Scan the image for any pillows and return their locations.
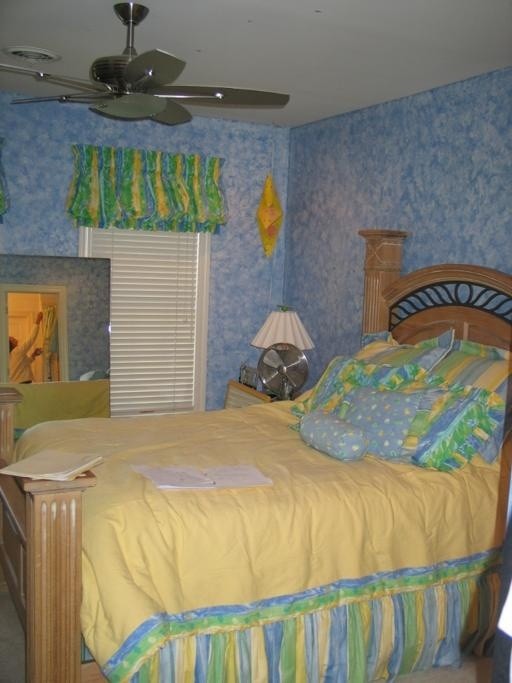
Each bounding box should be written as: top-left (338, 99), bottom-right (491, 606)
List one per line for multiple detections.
top-left (295, 328), bottom-right (511, 474)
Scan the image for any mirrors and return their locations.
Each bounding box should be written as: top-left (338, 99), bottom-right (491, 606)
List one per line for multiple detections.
top-left (0, 283), bottom-right (69, 384)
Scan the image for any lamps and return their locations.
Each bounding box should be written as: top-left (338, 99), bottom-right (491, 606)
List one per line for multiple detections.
top-left (249, 305), bottom-right (316, 353)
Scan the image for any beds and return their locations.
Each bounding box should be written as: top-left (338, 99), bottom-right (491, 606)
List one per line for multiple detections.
top-left (0, 229), bottom-right (511, 683)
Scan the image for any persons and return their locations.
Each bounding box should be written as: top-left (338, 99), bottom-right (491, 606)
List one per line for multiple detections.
top-left (7, 312), bottom-right (44, 386)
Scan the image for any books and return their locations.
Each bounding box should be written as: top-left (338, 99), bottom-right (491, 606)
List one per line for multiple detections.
top-left (0, 447), bottom-right (104, 483)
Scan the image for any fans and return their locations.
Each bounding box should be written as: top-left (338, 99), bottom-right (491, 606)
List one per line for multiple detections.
top-left (0, 3), bottom-right (292, 127)
top-left (256, 343), bottom-right (308, 398)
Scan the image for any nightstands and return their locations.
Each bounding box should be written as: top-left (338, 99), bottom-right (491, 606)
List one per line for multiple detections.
top-left (222, 381), bottom-right (302, 410)
